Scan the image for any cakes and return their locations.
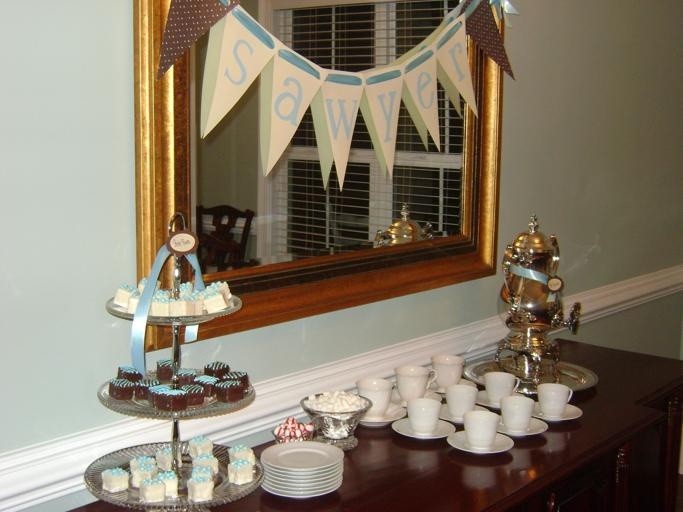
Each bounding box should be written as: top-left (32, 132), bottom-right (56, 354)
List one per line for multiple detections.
top-left (274, 418), bottom-right (310, 443)
top-left (108, 360), bottom-right (249, 411)
top-left (100, 436), bottom-right (219, 503)
top-left (228, 459), bottom-right (254, 485)
top-left (228, 445), bottom-right (256, 465)
top-left (114, 279), bottom-right (232, 316)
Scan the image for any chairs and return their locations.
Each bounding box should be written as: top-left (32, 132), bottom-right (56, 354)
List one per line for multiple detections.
top-left (196, 204), bottom-right (256, 276)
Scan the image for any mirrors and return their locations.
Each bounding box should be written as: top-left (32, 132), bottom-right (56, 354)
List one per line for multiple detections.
top-left (132, 0), bottom-right (506, 352)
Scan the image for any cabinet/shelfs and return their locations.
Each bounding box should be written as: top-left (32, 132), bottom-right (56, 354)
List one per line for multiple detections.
top-left (62, 336), bottom-right (682, 512)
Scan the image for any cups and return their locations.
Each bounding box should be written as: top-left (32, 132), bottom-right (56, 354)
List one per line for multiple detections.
top-left (351, 354), bottom-right (584, 457)
top-left (297, 390), bottom-right (373, 453)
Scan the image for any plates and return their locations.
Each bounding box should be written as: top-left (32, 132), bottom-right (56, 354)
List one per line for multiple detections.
top-left (258, 438), bottom-right (346, 501)
